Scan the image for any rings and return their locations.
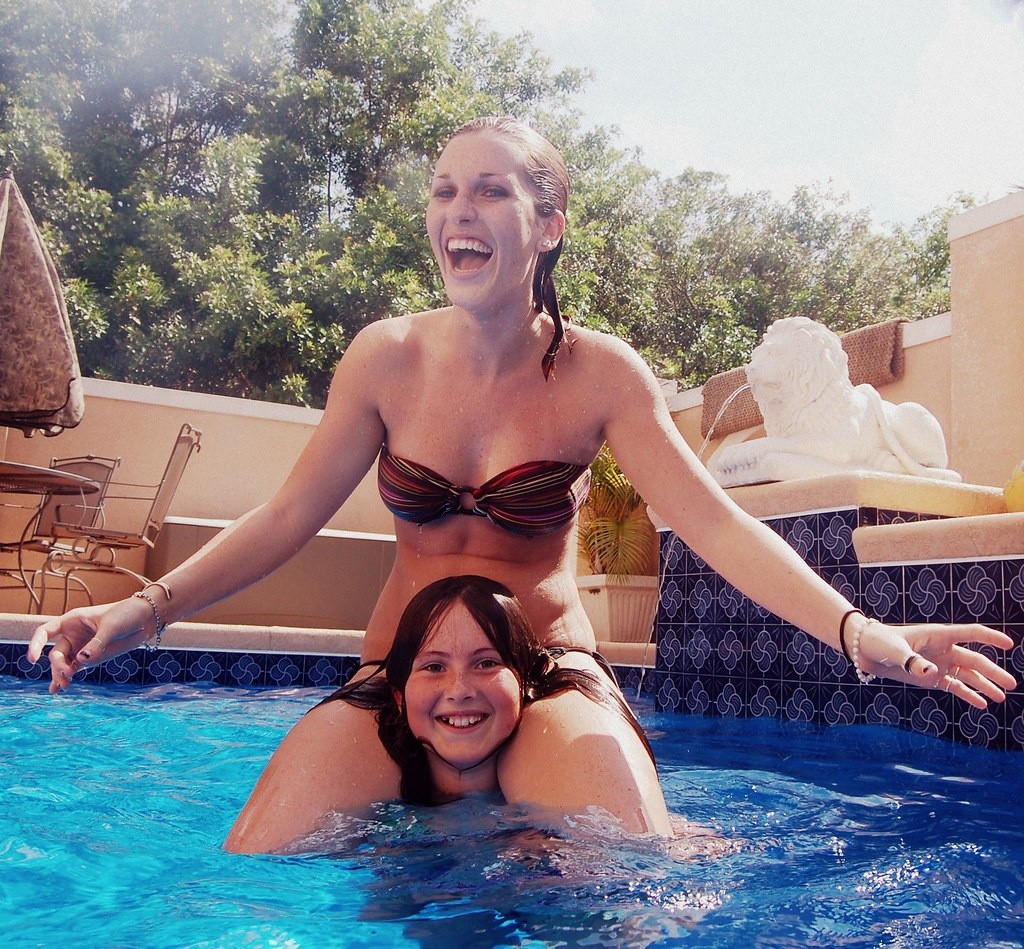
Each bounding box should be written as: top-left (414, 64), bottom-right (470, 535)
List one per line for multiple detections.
top-left (91, 637), bottom-right (106, 653)
top-left (900, 651), bottom-right (922, 675)
top-left (953, 664), bottom-right (962, 680)
top-left (946, 678), bottom-right (955, 692)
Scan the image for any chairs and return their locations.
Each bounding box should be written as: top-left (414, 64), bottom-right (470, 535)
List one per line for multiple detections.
top-left (0, 453), bottom-right (121, 614)
top-left (35, 423), bottom-right (204, 618)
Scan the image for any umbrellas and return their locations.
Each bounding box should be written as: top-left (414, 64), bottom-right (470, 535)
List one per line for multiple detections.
top-left (1, 174), bottom-right (86, 437)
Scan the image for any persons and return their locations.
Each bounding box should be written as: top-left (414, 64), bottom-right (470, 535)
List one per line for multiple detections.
top-left (305, 576), bottom-right (656, 797)
top-left (26, 105), bottom-right (1016, 853)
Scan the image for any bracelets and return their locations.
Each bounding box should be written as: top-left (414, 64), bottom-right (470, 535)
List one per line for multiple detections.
top-left (853, 617), bottom-right (881, 683)
top-left (840, 607), bottom-right (864, 664)
top-left (130, 590), bottom-right (160, 652)
top-left (142, 581), bottom-right (172, 634)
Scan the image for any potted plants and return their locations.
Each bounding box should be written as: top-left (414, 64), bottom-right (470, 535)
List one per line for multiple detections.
top-left (578, 442), bottom-right (660, 644)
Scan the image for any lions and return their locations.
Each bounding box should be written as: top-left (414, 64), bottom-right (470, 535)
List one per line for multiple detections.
top-left (744, 316), bottom-right (965, 481)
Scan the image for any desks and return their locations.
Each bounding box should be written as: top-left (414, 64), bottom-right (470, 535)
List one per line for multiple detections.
top-left (0, 460), bottom-right (101, 615)
